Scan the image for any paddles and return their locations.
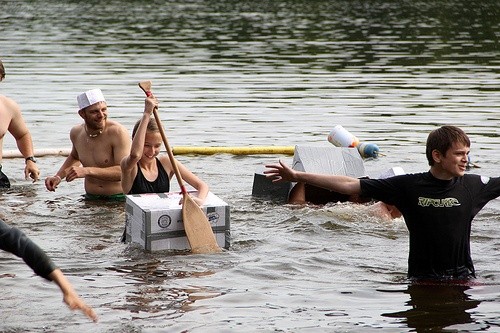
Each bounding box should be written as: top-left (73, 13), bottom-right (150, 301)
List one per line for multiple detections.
top-left (138, 81), bottom-right (221, 252)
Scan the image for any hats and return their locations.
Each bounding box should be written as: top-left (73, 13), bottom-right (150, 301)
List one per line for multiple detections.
top-left (76, 89), bottom-right (105, 111)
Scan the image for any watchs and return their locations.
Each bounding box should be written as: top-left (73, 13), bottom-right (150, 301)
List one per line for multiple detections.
top-left (26, 157), bottom-right (37, 163)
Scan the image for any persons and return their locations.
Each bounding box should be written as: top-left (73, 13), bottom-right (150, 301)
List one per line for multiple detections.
top-left (0, 218), bottom-right (98, 322)
top-left (287, 180), bottom-right (403, 220)
top-left (264, 126), bottom-right (500, 281)
top-left (0, 58), bottom-right (39, 192)
top-left (118, 95), bottom-right (209, 243)
top-left (45, 89), bottom-right (131, 204)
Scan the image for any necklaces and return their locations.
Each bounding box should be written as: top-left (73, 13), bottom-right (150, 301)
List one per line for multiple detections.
top-left (84, 120), bottom-right (105, 137)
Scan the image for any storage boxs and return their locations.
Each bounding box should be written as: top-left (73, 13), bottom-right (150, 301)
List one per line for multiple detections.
top-left (126, 191), bottom-right (230, 252)
top-left (380, 167), bottom-right (404, 179)
top-left (252, 173), bottom-right (299, 201)
top-left (292, 146), bottom-right (370, 203)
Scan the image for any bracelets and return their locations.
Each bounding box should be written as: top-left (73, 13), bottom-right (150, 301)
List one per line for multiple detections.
top-left (54, 175), bottom-right (62, 182)
